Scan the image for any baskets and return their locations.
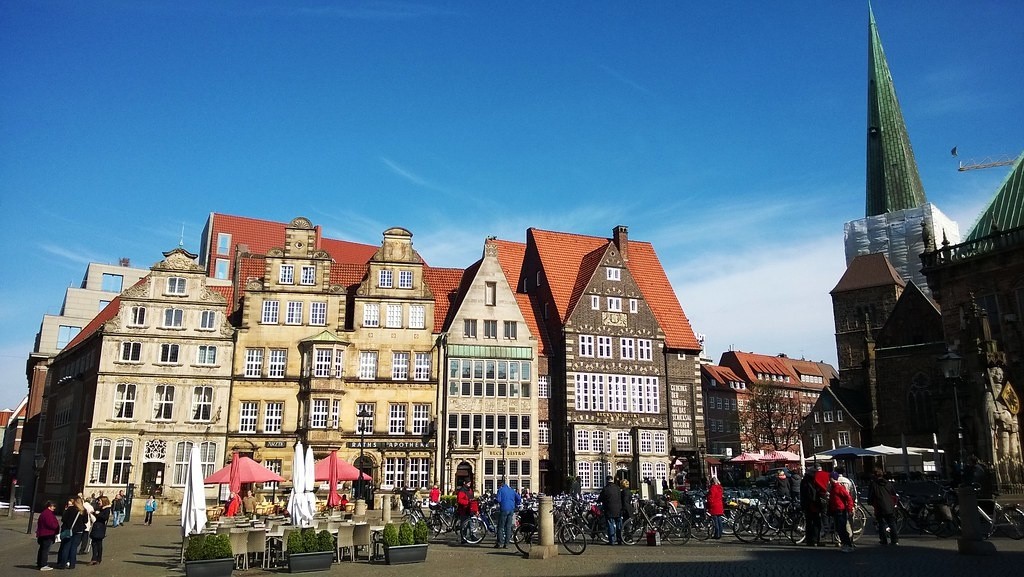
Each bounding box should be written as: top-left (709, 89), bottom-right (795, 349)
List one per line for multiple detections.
top-left (428, 503), bottom-right (441, 510)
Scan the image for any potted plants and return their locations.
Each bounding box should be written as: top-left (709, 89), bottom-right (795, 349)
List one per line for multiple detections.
top-left (382, 520), bottom-right (429, 565)
top-left (284, 528), bottom-right (334, 572)
top-left (184, 533), bottom-right (235, 577)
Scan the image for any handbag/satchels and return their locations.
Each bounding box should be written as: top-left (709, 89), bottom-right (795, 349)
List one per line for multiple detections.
top-left (815, 496), bottom-right (827, 513)
top-left (845, 504), bottom-right (855, 519)
top-left (60, 529), bottom-right (72, 540)
top-left (470, 501), bottom-right (478, 511)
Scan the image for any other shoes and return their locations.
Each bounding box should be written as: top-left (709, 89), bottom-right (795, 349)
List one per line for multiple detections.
top-left (625, 540), bottom-right (636, 544)
top-left (879, 541), bottom-right (899, 546)
top-left (711, 536), bottom-right (721, 540)
top-left (88, 560), bottom-right (101, 566)
top-left (493, 544), bottom-right (507, 548)
top-left (78, 551), bottom-right (87, 555)
top-left (55, 562), bottom-right (75, 569)
top-left (113, 523), bottom-right (124, 528)
top-left (461, 536), bottom-right (478, 544)
top-left (607, 541), bottom-right (622, 546)
top-left (40, 565), bottom-right (53, 571)
top-left (144, 523), bottom-right (151, 526)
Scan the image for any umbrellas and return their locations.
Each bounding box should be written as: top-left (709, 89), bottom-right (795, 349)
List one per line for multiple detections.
top-left (805, 443), bottom-right (923, 478)
top-left (180, 436), bottom-right (373, 571)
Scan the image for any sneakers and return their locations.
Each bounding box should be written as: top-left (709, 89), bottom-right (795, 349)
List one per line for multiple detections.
top-left (840, 543), bottom-right (855, 553)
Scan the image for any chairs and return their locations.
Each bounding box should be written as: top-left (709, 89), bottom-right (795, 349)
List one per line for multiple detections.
top-left (854, 471), bottom-right (937, 484)
top-left (181, 502), bottom-right (382, 571)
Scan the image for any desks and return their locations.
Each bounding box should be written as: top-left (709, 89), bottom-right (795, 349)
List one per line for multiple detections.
top-left (266, 526), bottom-right (384, 563)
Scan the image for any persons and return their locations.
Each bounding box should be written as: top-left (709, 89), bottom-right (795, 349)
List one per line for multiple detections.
top-left (489, 481), bottom-right (520, 550)
top-left (400, 486), bottom-right (411, 504)
top-left (243, 488), bottom-right (256, 517)
top-left (706, 478), bottom-right (725, 540)
top-left (775, 462), bottom-right (902, 552)
top-left (596, 476), bottom-right (623, 546)
top-left (619, 479), bottom-right (634, 546)
top-left (457, 477), bottom-right (480, 544)
top-left (341, 495), bottom-right (349, 512)
top-left (430, 485), bottom-right (439, 504)
top-left (985, 367), bottom-right (1023, 484)
top-left (36, 490), bottom-right (156, 572)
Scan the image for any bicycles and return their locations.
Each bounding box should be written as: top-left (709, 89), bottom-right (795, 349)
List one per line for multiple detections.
top-left (399, 471), bottom-right (1024, 548)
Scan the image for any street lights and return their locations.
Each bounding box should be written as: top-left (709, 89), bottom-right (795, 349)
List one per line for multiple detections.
top-left (26, 453), bottom-right (47, 534)
top-left (356, 403), bottom-right (373, 499)
top-left (936, 345), bottom-right (965, 482)
top-left (599, 450), bottom-right (606, 486)
top-left (699, 442), bottom-right (708, 489)
top-left (500, 432), bottom-right (509, 482)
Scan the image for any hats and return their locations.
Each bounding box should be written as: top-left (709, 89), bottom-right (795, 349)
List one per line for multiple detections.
top-left (620, 479), bottom-right (629, 488)
top-left (830, 471), bottom-right (839, 480)
top-left (779, 471), bottom-right (784, 476)
